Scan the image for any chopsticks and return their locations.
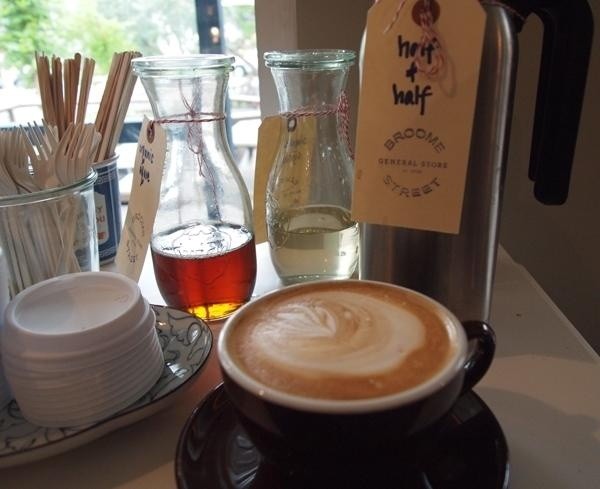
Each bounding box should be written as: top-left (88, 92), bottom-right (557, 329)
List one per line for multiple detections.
top-left (33, 50), bottom-right (141, 164)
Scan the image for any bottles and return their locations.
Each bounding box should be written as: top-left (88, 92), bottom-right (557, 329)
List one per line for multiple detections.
top-left (357, 1), bottom-right (514, 323)
top-left (129, 56), bottom-right (256, 323)
top-left (263, 50), bottom-right (363, 285)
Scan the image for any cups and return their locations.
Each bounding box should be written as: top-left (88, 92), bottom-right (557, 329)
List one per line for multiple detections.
top-left (216, 277), bottom-right (497, 487)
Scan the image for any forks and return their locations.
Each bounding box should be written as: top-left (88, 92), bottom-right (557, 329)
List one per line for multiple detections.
top-left (0, 118), bottom-right (101, 297)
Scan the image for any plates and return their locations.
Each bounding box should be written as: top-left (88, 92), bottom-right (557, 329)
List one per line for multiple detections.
top-left (1, 300), bottom-right (212, 472)
top-left (0, 271), bottom-right (166, 429)
top-left (173, 380), bottom-right (503, 486)
